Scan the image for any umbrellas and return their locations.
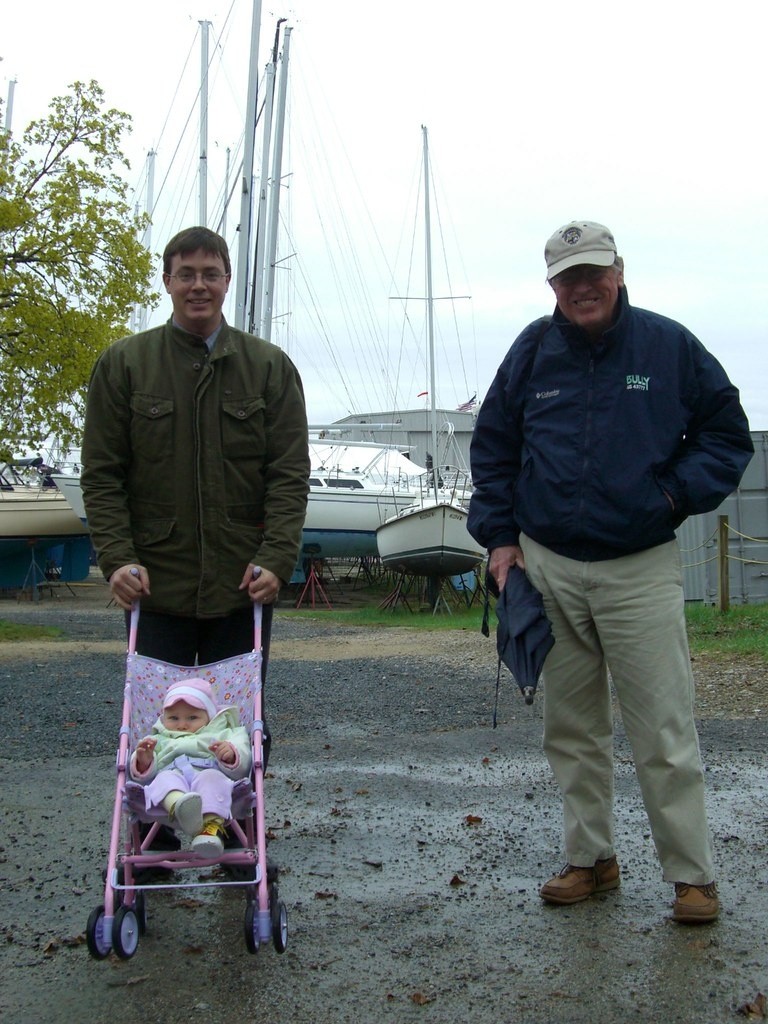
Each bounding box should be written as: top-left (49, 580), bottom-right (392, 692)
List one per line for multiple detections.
top-left (483, 554), bottom-right (554, 703)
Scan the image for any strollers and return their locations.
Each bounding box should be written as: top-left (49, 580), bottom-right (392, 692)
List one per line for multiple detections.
top-left (84, 560), bottom-right (292, 959)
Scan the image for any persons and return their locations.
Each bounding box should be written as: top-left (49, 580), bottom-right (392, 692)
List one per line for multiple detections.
top-left (466, 220), bottom-right (756, 923)
top-left (130, 678), bottom-right (251, 859)
top-left (80, 227), bottom-right (311, 887)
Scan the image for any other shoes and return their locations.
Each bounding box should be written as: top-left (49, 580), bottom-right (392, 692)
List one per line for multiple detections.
top-left (102, 863), bottom-right (159, 886)
top-left (191, 834), bottom-right (222, 857)
top-left (175, 791), bottom-right (204, 836)
top-left (220, 848), bottom-right (278, 883)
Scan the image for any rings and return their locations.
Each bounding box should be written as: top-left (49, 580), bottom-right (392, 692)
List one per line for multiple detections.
top-left (264, 597), bottom-right (267, 600)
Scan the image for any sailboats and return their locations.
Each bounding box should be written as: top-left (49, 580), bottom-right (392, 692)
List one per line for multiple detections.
top-left (371, 115), bottom-right (490, 585)
top-left (0, 0), bottom-right (454, 569)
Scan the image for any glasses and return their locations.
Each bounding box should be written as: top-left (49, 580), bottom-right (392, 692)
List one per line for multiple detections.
top-left (167, 269), bottom-right (229, 285)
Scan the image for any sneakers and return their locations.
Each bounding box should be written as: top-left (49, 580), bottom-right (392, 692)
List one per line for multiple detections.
top-left (538, 853), bottom-right (620, 903)
top-left (672, 880), bottom-right (720, 922)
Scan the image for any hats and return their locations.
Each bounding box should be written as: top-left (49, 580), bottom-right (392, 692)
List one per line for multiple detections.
top-left (545, 220), bottom-right (617, 281)
top-left (163, 678), bottom-right (218, 722)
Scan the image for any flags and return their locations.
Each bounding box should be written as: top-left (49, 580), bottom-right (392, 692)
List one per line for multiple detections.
top-left (458, 395), bottom-right (476, 412)
